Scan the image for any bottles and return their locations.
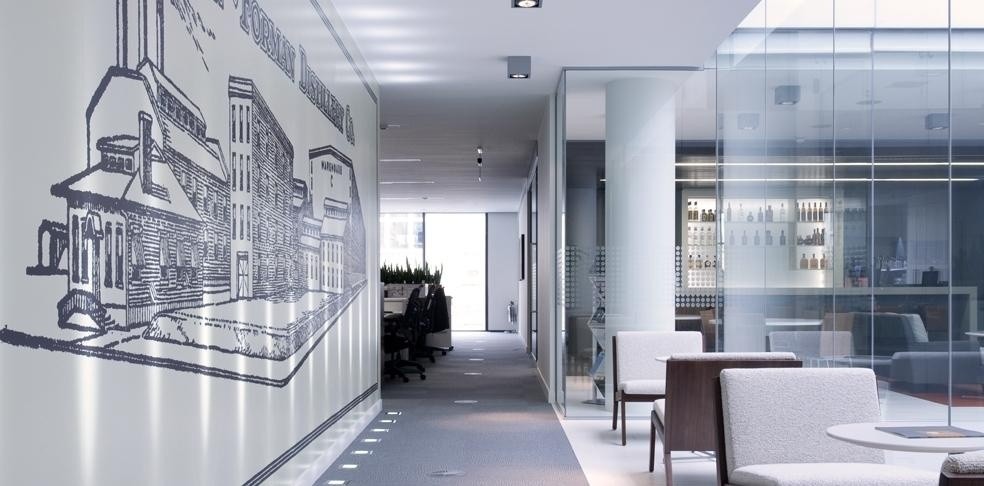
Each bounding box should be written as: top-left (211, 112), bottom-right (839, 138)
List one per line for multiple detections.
top-left (737, 202), bottom-right (787, 223)
top-left (780, 230), bottom-right (787, 245)
top-left (726, 201), bottom-right (732, 221)
top-left (765, 230), bottom-right (773, 246)
top-left (797, 228), bottom-right (825, 247)
top-left (796, 202), bottom-right (831, 222)
top-left (688, 254), bottom-right (717, 269)
top-left (741, 230), bottom-right (748, 245)
top-left (688, 226), bottom-right (717, 245)
top-left (687, 201), bottom-right (718, 222)
top-left (799, 253), bottom-right (828, 269)
top-left (728, 228), bottom-right (736, 246)
top-left (753, 230), bottom-right (761, 245)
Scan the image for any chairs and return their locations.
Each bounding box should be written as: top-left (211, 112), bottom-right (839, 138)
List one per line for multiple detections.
top-left (380, 284), bottom-right (454, 382)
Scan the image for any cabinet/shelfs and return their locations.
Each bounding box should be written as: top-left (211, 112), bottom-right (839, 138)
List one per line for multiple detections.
top-left (681, 188), bottom-right (844, 286)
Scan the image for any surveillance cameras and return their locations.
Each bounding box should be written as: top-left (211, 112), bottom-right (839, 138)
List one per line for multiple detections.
top-left (476, 155), bottom-right (483, 166)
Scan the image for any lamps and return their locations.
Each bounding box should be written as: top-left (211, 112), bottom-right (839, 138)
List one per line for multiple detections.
top-left (775, 86), bottom-right (800, 105)
top-left (924, 114), bottom-right (949, 131)
top-left (738, 115), bottom-right (758, 130)
top-left (507, 56), bottom-right (531, 79)
top-left (511, 0), bottom-right (542, 8)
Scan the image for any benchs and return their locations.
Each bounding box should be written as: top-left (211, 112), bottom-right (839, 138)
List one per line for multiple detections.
top-left (611, 309), bottom-right (984, 486)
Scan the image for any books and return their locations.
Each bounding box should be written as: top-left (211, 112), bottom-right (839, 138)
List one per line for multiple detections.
top-left (875, 425), bottom-right (984, 439)
